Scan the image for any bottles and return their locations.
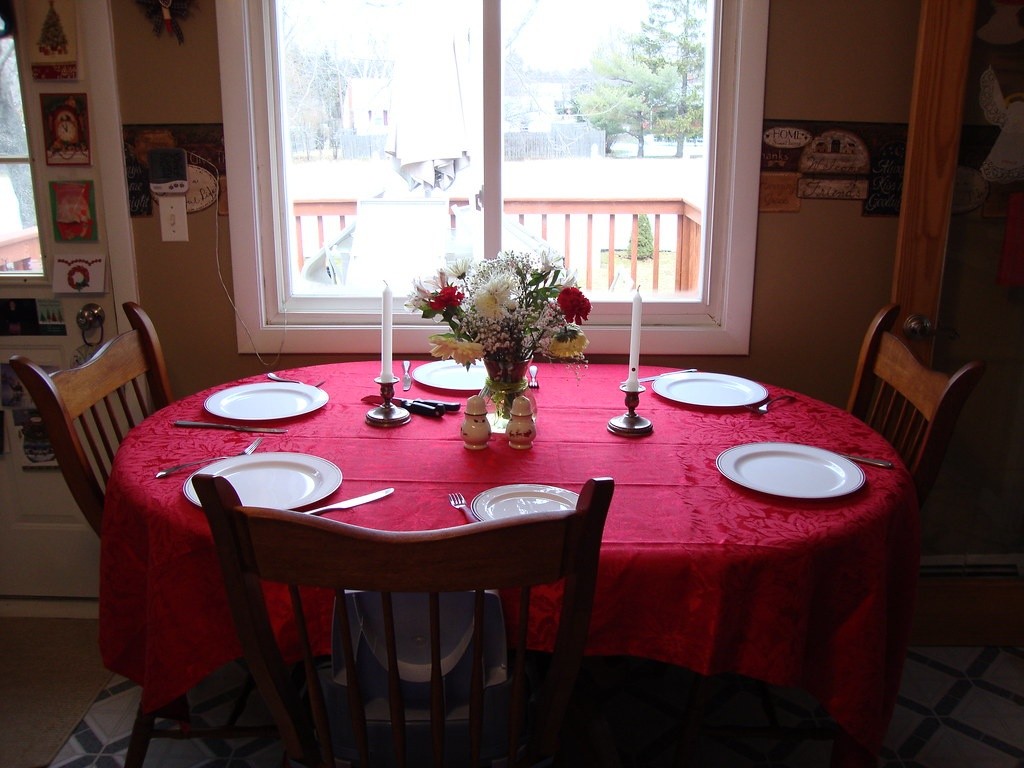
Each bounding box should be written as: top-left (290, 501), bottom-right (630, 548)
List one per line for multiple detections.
top-left (460, 396), bottom-right (491, 450)
top-left (506, 397), bottom-right (536, 450)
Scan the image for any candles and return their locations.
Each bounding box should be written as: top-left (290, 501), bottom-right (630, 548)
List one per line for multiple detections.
top-left (628, 286), bottom-right (641, 391)
top-left (380, 280), bottom-right (393, 382)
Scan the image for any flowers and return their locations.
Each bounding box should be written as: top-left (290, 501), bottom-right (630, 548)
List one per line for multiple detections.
top-left (402, 246), bottom-right (591, 420)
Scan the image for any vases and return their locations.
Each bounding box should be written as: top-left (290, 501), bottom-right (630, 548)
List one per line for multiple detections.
top-left (478, 377), bottom-right (538, 433)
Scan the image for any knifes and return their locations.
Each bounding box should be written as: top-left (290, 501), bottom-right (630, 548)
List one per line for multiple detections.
top-left (304, 488), bottom-right (394, 515)
top-left (620, 369), bottom-right (698, 384)
top-left (175, 421), bottom-right (288, 432)
top-left (403, 361), bottom-right (411, 390)
top-left (361, 395), bottom-right (460, 418)
top-left (825, 449), bottom-right (893, 469)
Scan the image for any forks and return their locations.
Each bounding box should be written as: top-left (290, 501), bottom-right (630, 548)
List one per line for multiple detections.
top-left (155, 438), bottom-right (263, 478)
top-left (268, 373), bottom-right (325, 387)
top-left (449, 493), bottom-right (479, 523)
top-left (745, 396), bottom-right (795, 414)
top-left (530, 366), bottom-right (538, 387)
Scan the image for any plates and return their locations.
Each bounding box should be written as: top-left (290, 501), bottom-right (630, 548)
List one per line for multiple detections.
top-left (204, 382), bottom-right (329, 421)
top-left (183, 452), bottom-right (342, 510)
top-left (716, 443), bottom-right (866, 498)
top-left (471, 484), bottom-right (579, 522)
top-left (412, 358), bottom-right (489, 390)
top-left (652, 372), bottom-right (768, 406)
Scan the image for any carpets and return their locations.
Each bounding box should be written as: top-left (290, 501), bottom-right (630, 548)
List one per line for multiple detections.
top-left (0, 617), bottom-right (118, 768)
top-left (46, 641), bottom-right (1024, 768)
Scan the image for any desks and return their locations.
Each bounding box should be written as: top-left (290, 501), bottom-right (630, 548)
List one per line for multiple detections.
top-left (100, 360), bottom-right (922, 768)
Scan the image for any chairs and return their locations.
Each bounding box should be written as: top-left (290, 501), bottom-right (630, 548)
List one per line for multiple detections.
top-left (9, 303), bottom-right (313, 768)
top-left (192, 474), bottom-right (615, 768)
top-left (848, 303), bottom-right (983, 500)
